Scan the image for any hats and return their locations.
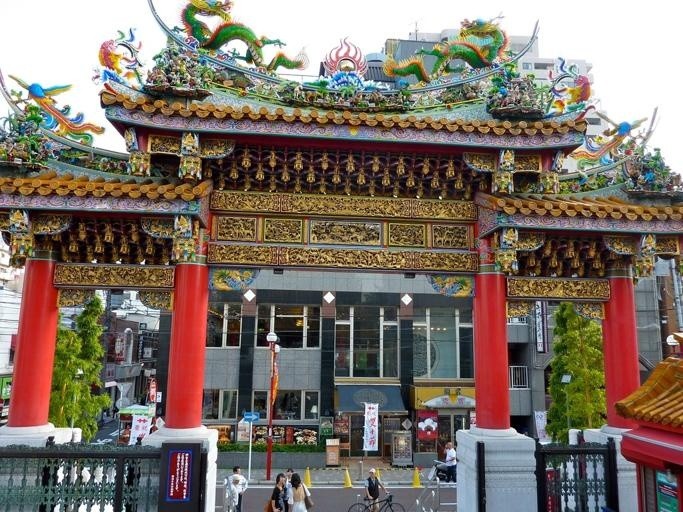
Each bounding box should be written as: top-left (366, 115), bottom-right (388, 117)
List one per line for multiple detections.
top-left (369, 468), bottom-right (376, 473)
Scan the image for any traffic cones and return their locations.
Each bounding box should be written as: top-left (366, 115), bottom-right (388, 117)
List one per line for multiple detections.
top-left (411, 465), bottom-right (422, 488)
top-left (344, 467), bottom-right (352, 488)
top-left (375, 467), bottom-right (380, 477)
top-left (303, 466), bottom-right (313, 488)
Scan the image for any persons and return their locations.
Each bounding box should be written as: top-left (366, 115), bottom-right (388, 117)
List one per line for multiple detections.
top-left (272, 468), bottom-right (311, 512)
top-left (443, 442), bottom-right (456, 482)
top-left (364, 468), bottom-right (388, 512)
top-left (122, 424), bottom-right (131, 441)
top-left (224, 466), bottom-right (248, 512)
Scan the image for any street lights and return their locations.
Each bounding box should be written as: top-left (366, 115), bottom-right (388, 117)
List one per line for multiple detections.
top-left (104, 381), bottom-right (123, 439)
top-left (266, 330), bottom-right (280, 480)
top-left (665, 334), bottom-right (679, 354)
top-left (560, 373), bottom-right (572, 430)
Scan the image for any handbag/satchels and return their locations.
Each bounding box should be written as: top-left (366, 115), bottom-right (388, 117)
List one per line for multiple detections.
top-left (264, 499), bottom-right (273, 512)
top-left (305, 496), bottom-right (314, 508)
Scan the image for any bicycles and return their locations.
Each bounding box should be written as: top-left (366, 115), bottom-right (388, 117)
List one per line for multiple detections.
top-left (347, 492), bottom-right (406, 511)
